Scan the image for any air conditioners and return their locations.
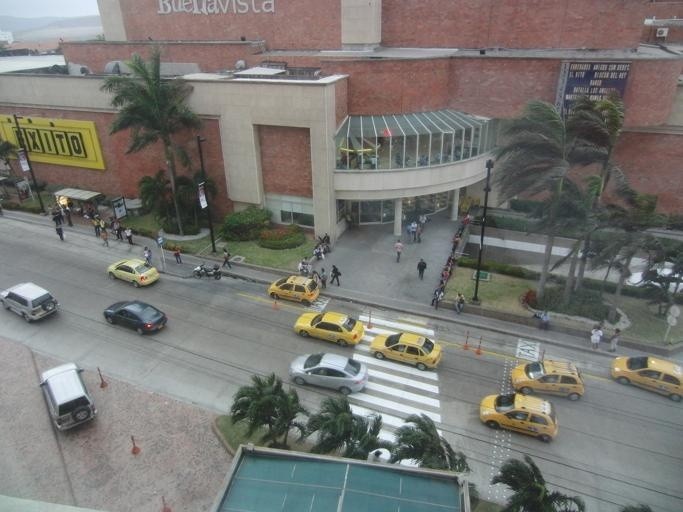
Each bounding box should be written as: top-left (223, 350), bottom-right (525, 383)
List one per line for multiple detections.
top-left (655, 27), bottom-right (669, 38)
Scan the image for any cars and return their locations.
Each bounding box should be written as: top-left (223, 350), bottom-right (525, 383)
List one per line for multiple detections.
top-left (104, 299), bottom-right (167, 337)
top-left (292, 311), bottom-right (365, 346)
top-left (513, 359), bottom-right (586, 401)
top-left (614, 355), bottom-right (683, 401)
top-left (478, 391), bottom-right (560, 444)
top-left (371, 332), bottom-right (442, 372)
top-left (266, 276), bottom-right (319, 304)
top-left (289, 352), bottom-right (368, 396)
top-left (624, 266), bottom-right (683, 295)
top-left (108, 260), bottom-right (158, 289)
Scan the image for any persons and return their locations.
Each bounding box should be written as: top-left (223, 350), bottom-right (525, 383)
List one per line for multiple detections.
top-left (539, 310), bottom-right (550, 330)
top-left (143, 246), bottom-right (153, 266)
top-left (396, 153), bottom-right (402, 166)
top-left (420, 154), bottom-right (425, 166)
top-left (221, 248), bottom-right (233, 269)
top-left (607, 327), bottom-right (621, 352)
top-left (173, 245), bottom-right (183, 264)
top-left (393, 212), bottom-right (470, 315)
top-left (50, 201), bottom-right (134, 249)
top-left (589, 325), bottom-right (604, 350)
top-left (341, 147), bottom-right (379, 169)
top-left (298, 232), bottom-right (342, 289)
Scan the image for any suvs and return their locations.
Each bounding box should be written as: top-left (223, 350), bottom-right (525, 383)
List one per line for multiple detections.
top-left (41, 363), bottom-right (98, 436)
top-left (0, 280), bottom-right (60, 323)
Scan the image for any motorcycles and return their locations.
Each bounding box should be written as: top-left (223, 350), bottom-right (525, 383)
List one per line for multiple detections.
top-left (193, 261), bottom-right (223, 280)
